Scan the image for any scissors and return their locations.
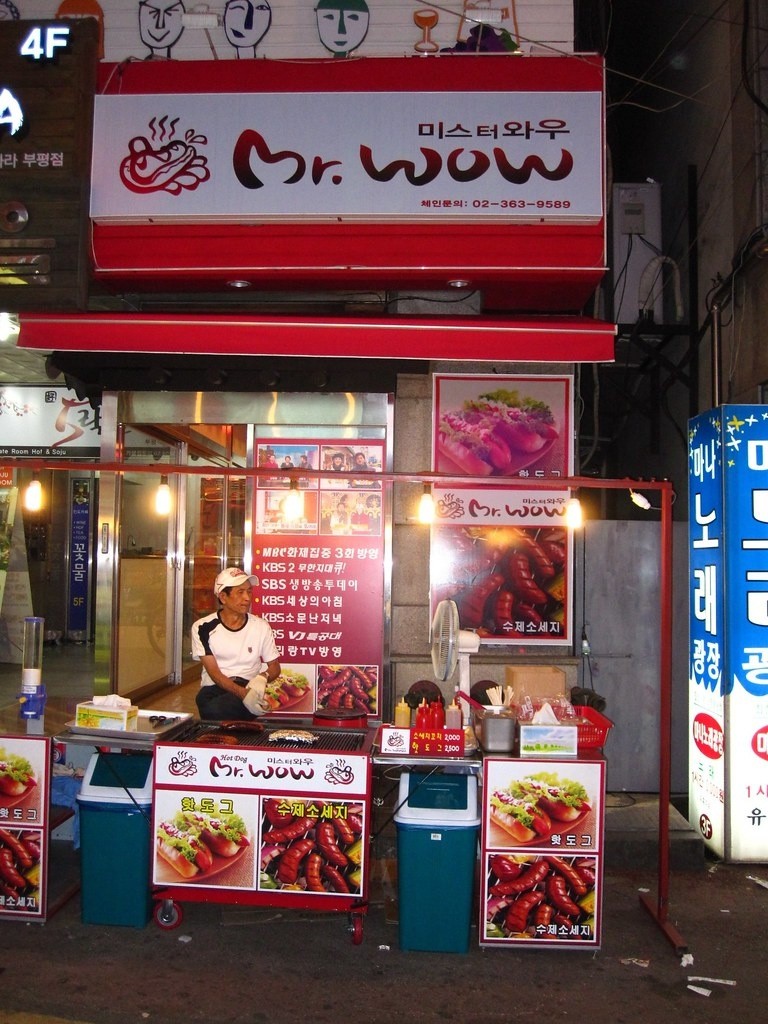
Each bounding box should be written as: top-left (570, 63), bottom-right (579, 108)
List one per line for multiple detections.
top-left (149, 715), bottom-right (166, 729)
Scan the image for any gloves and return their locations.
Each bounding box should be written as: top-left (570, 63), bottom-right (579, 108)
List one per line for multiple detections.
top-left (245, 675), bottom-right (267, 698)
top-left (242, 689), bottom-right (272, 716)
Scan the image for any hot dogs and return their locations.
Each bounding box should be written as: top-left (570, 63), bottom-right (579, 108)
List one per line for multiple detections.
top-left (489, 772), bottom-right (593, 842)
top-left (436, 389), bottom-right (558, 476)
top-left (0, 747), bottom-right (39, 797)
top-left (260, 669), bottom-right (311, 711)
top-left (156, 811), bottom-right (250, 877)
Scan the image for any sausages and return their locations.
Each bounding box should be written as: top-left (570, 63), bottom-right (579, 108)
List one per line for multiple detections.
top-left (316, 664), bottom-right (376, 714)
top-left (439, 530), bottom-right (568, 637)
top-left (488, 854), bottom-right (595, 939)
top-left (0, 828), bottom-right (32, 910)
top-left (260, 802), bottom-right (353, 894)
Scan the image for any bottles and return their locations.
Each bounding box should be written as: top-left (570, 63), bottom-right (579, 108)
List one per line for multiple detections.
top-left (446, 699), bottom-right (461, 729)
top-left (394, 696), bottom-right (411, 728)
top-left (431, 693), bottom-right (444, 729)
top-left (414, 698), bottom-right (431, 728)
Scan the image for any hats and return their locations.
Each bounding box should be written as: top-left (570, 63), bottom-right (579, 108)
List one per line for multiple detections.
top-left (214, 567), bottom-right (260, 598)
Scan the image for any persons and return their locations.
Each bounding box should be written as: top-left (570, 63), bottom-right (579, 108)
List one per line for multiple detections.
top-left (350, 453), bottom-right (376, 471)
top-left (264, 455), bottom-right (278, 468)
top-left (351, 505), bottom-right (369, 534)
top-left (191, 568), bottom-right (281, 721)
top-left (329, 504), bottom-right (350, 534)
top-left (281, 456), bottom-right (294, 468)
top-left (326, 451), bottom-right (348, 471)
top-left (299, 455), bottom-right (312, 470)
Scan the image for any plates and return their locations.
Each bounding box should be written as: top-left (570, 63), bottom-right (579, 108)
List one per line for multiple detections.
top-left (158, 820), bottom-right (247, 882)
top-left (495, 791), bottom-right (592, 847)
top-left (270, 687), bottom-right (310, 710)
top-left (0, 770), bottom-right (37, 808)
top-left (441, 404), bottom-right (557, 477)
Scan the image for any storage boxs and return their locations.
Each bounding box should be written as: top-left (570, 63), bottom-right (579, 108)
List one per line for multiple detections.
top-left (505, 663), bottom-right (567, 705)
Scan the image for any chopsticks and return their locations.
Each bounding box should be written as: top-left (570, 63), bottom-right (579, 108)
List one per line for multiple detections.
top-left (486, 685), bottom-right (514, 705)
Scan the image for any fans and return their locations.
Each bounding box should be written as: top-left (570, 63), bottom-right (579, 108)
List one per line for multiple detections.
top-left (429, 599), bottom-right (482, 727)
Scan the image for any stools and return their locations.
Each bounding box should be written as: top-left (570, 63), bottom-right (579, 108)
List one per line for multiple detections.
top-left (311, 707), bottom-right (369, 728)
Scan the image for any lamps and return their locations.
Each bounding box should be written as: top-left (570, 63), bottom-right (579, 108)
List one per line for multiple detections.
top-left (582, 631), bottom-right (591, 657)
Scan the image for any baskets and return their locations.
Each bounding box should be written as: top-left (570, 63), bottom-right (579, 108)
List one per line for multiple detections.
top-left (512, 703), bottom-right (614, 747)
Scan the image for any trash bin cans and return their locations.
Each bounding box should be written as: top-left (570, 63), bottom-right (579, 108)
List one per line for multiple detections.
top-left (394, 771), bottom-right (482, 955)
top-left (74, 752), bottom-right (153, 929)
